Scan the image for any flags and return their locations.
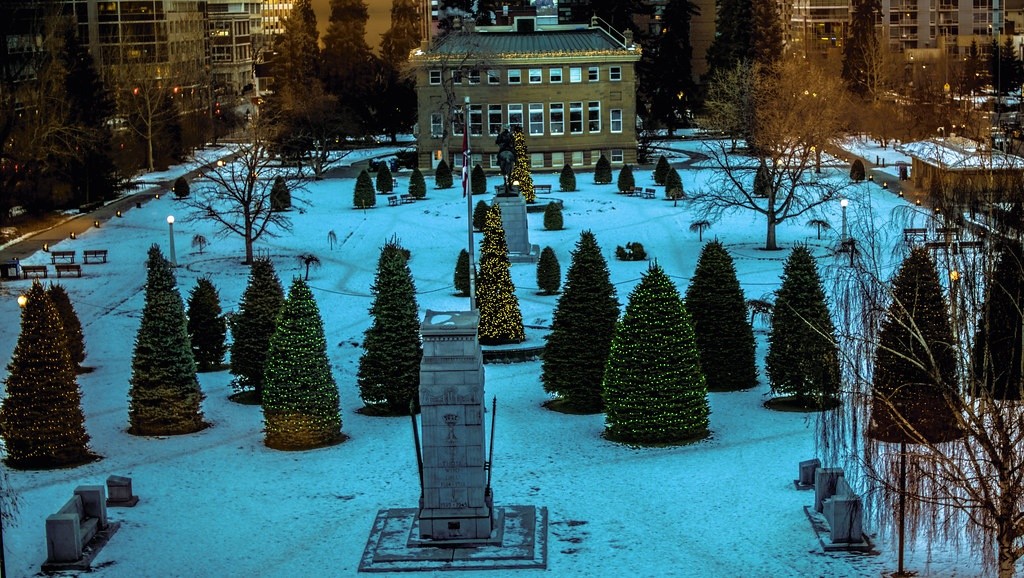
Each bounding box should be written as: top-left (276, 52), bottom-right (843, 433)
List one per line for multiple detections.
top-left (460, 97), bottom-right (470, 200)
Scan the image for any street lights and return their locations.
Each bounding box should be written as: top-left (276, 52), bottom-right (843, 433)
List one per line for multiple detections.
top-left (840, 198), bottom-right (848, 242)
top-left (167, 215), bottom-right (178, 266)
top-left (17, 296), bottom-right (27, 317)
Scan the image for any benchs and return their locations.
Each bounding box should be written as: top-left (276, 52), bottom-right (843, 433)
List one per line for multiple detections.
top-left (55, 264), bottom-right (82, 277)
top-left (22, 266), bottom-right (47, 279)
top-left (401, 194), bottom-right (416, 203)
top-left (642, 188), bottom-right (655, 199)
top-left (625, 186), bottom-right (642, 197)
top-left (388, 196), bottom-right (400, 205)
top-left (82, 250), bottom-right (107, 264)
top-left (805, 467), bottom-right (871, 552)
top-left (41, 484), bottom-right (120, 574)
top-left (533, 184), bottom-right (551, 194)
top-left (51, 251), bottom-right (75, 264)
